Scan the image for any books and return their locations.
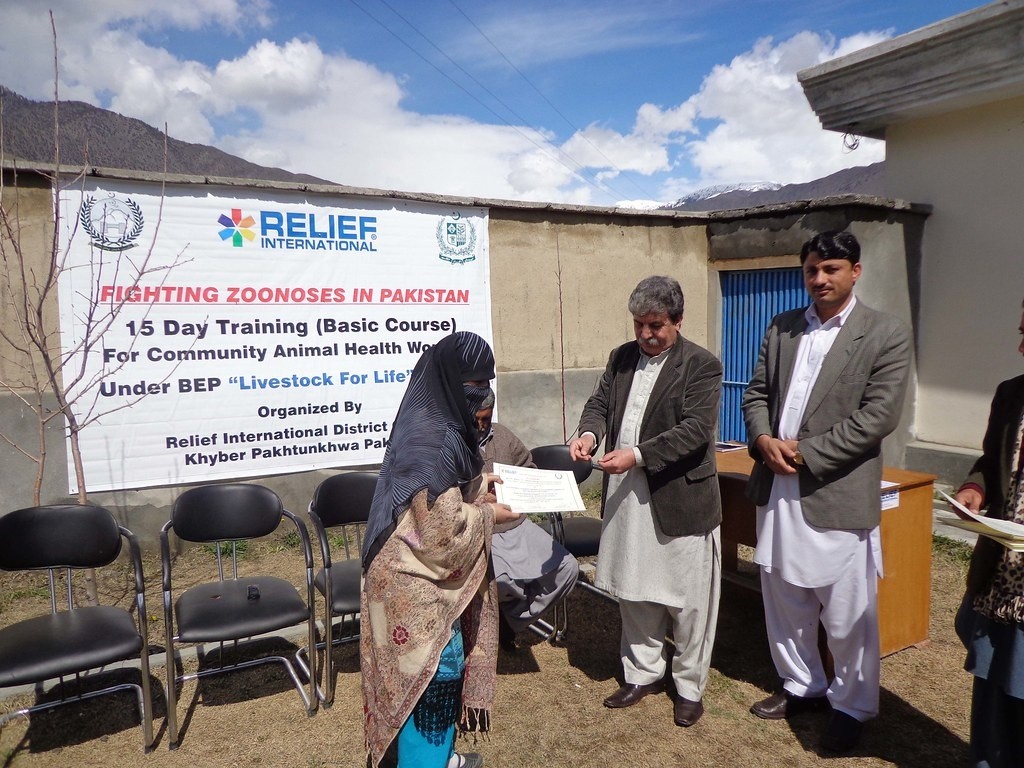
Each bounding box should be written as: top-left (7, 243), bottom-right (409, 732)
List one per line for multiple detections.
top-left (935, 489), bottom-right (1023, 553)
top-left (713, 441), bottom-right (748, 453)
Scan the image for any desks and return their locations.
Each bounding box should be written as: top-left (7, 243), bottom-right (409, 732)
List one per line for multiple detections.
top-left (716, 440), bottom-right (937, 664)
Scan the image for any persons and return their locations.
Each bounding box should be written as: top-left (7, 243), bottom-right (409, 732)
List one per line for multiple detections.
top-left (950, 301), bottom-right (1024, 768)
top-left (476, 388), bottom-right (579, 657)
top-left (741, 231), bottom-right (911, 752)
top-left (360, 331), bottom-right (521, 767)
top-left (570, 274), bottom-right (722, 727)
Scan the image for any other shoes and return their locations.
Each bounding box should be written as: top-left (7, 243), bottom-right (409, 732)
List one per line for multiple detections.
top-left (454, 752), bottom-right (483, 768)
top-left (499, 604), bottom-right (516, 653)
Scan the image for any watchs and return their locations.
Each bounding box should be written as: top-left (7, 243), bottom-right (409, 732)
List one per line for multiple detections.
top-left (793, 447), bottom-right (807, 466)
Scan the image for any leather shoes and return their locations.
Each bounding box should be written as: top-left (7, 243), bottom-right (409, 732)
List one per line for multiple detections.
top-left (604, 679), bottom-right (665, 707)
top-left (751, 690), bottom-right (824, 719)
top-left (674, 695), bottom-right (704, 726)
top-left (820, 708), bottom-right (866, 751)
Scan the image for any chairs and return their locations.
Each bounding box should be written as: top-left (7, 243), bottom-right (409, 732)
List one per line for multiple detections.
top-left (159, 485), bottom-right (320, 749)
top-left (526, 444), bottom-right (617, 643)
top-left (1, 504), bottom-right (156, 756)
top-left (308, 469), bottom-right (379, 706)
